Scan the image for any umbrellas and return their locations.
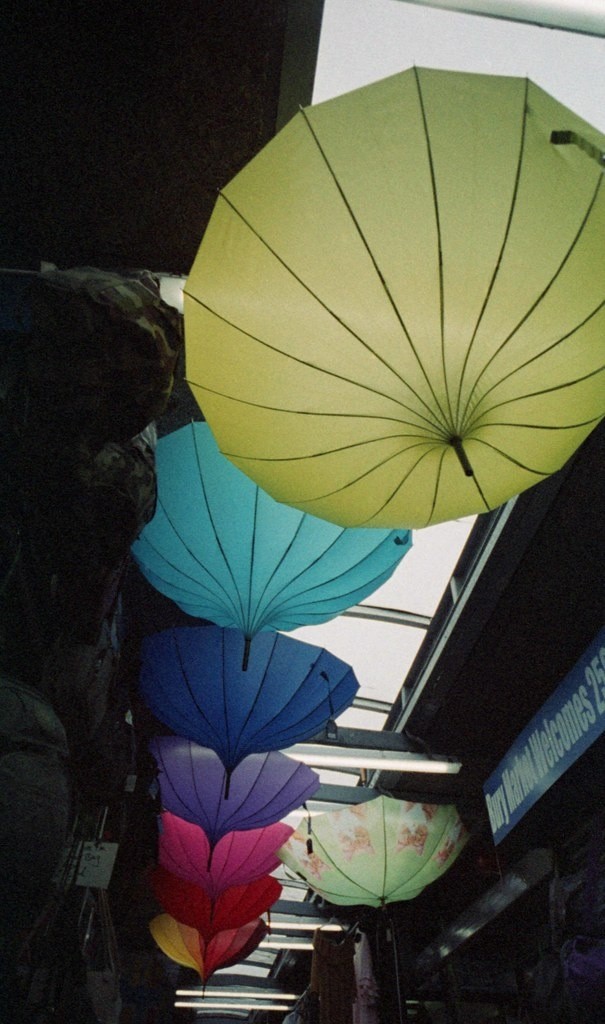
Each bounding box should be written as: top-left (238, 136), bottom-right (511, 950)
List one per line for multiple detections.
top-left (180, 65), bottom-right (605, 530)
top-left (129, 416), bottom-right (413, 672)
top-left (148, 913), bottom-right (272, 1000)
top-left (148, 862), bottom-right (283, 963)
top-left (139, 624), bottom-right (360, 800)
top-left (147, 735), bottom-right (321, 872)
top-left (155, 811), bottom-right (295, 923)
top-left (273, 793), bottom-right (470, 919)
